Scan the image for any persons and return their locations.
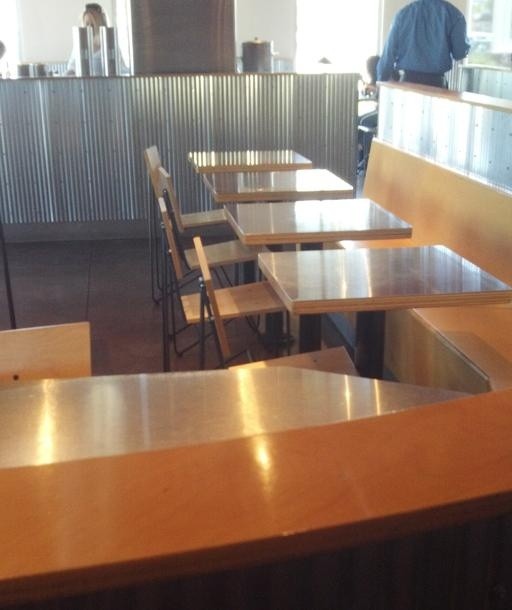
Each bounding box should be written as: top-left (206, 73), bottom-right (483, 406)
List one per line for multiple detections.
top-left (357, 55), bottom-right (401, 178)
top-left (375, 0), bottom-right (473, 90)
top-left (67, 4), bottom-right (131, 77)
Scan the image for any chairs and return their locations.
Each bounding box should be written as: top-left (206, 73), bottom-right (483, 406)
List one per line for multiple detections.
top-left (0, 322), bottom-right (92, 389)
top-left (145, 146), bottom-right (291, 371)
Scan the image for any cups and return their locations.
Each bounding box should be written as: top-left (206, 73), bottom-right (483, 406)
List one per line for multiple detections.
top-left (17, 63), bottom-right (46, 76)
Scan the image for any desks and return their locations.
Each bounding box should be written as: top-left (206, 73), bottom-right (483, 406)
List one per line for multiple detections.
top-left (0, 366), bottom-right (472, 467)
top-left (223, 198), bottom-right (412, 353)
top-left (257, 244), bottom-right (512, 379)
top-left (188, 150), bottom-right (313, 284)
top-left (200, 168), bottom-right (353, 344)
